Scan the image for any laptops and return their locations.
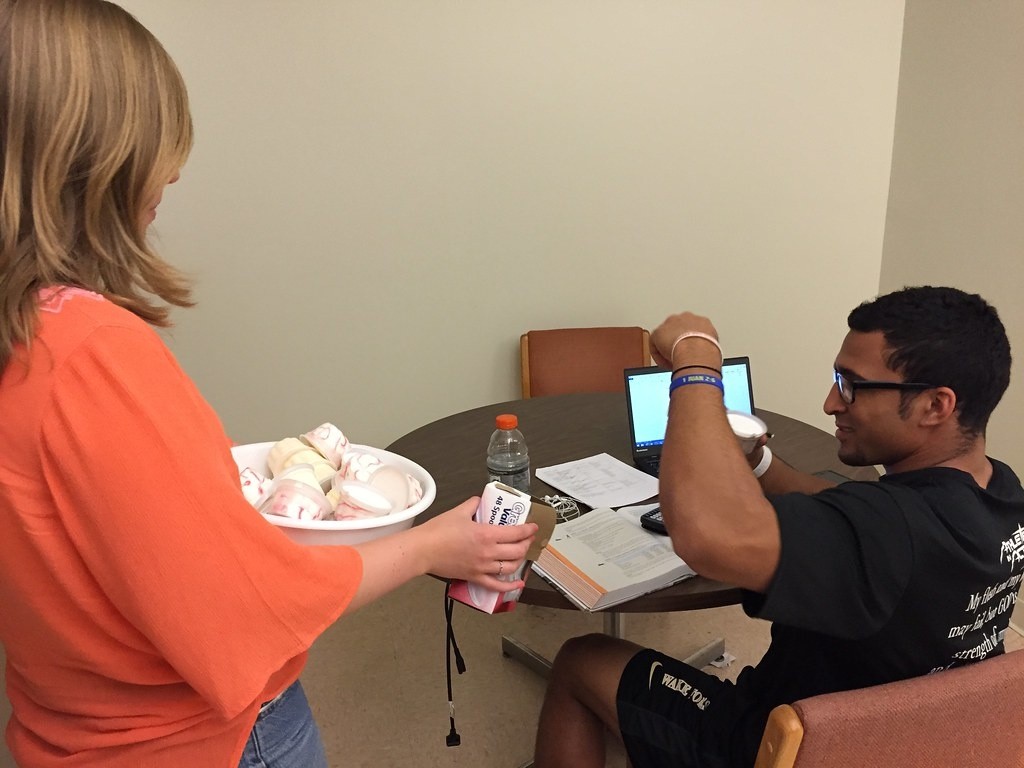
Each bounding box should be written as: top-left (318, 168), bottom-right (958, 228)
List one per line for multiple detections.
top-left (624, 357), bottom-right (756, 478)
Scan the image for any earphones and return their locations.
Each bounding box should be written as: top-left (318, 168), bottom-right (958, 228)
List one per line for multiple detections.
top-left (545, 495), bottom-right (552, 505)
top-left (553, 495), bottom-right (562, 502)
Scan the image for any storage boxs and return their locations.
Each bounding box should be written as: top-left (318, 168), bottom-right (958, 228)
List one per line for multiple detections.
top-left (447, 481), bottom-right (557, 614)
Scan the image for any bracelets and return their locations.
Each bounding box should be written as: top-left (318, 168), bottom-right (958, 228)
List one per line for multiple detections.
top-left (671, 330), bottom-right (725, 361)
top-left (746, 444), bottom-right (774, 485)
top-left (668, 363), bottom-right (727, 372)
top-left (663, 375), bottom-right (732, 394)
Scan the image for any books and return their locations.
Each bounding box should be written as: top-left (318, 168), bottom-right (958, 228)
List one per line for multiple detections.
top-left (534, 499), bottom-right (717, 611)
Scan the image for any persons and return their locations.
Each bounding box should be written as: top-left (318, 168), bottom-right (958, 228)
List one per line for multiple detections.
top-left (0, 0), bottom-right (540, 768)
top-left (539, 285), bottom-right (1024, 768)
top-left (534, 524), bottom-right (539, 526)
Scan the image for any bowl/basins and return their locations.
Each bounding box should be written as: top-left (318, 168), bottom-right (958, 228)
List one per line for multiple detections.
top-left (725, 410), bottom-right (766, 454)
top-left (231, 441), bottom-right (437, 544)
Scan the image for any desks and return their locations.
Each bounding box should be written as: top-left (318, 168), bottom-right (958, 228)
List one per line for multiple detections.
top-left (383, 399), bottom-right (880, 680)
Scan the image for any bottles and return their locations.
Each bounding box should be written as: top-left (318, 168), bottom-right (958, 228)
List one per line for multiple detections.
top-left (486, 414), bottom-right (532, 496)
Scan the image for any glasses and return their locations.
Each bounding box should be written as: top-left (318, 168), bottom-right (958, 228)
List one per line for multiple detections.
top-left (833, 369), bottom-right (962, 404)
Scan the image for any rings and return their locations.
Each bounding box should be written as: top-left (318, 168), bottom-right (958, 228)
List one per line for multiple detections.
top-left (496, 560), bottom-right (503, 575)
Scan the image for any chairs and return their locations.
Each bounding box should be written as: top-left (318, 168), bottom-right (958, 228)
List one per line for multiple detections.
top-left (520, 325), bottom-right (650, 399)
top-left (754, 647), bottom-right (1024, 768)
